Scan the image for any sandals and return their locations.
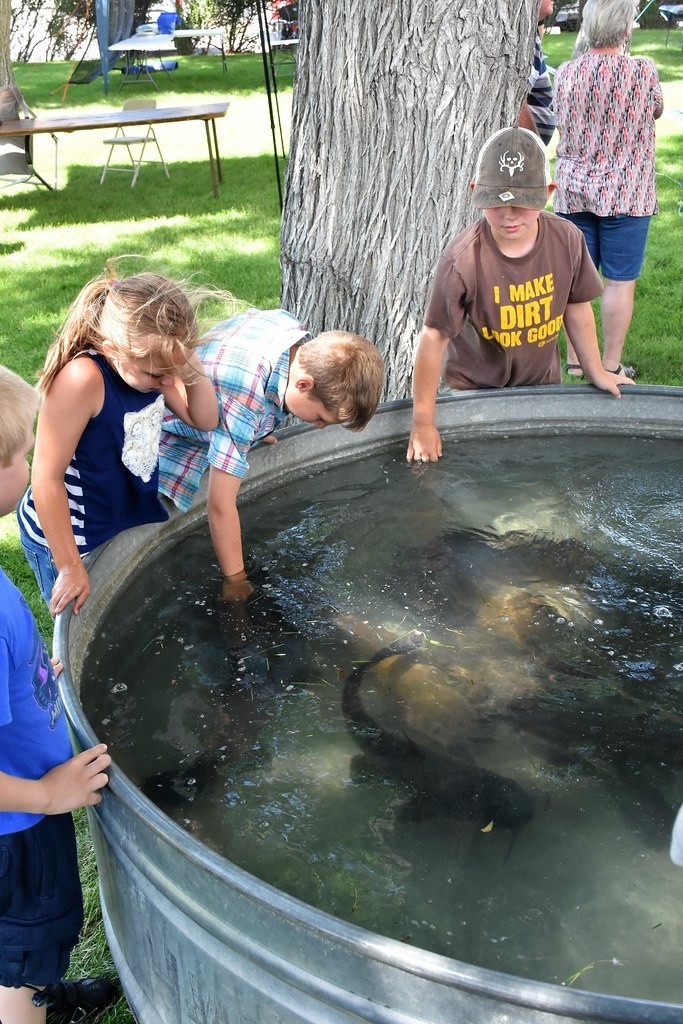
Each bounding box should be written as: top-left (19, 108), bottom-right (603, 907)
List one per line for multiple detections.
top-left (565, 364), bottom-right (585, 382)
top-left (603, 365), bottom-right (638, 381)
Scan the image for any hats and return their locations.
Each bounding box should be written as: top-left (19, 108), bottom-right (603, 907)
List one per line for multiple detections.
top-left (472, 125), bottom-right (555, 211)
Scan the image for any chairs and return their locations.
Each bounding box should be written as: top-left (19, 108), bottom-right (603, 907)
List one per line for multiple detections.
top-left (0, 83), bottom-right (58, 190)
top-left (100, 99), bottom-right (170, 188)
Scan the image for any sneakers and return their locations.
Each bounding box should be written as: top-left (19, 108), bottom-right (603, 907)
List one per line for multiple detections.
top-left (46, 974), bottom-right (118, 1024)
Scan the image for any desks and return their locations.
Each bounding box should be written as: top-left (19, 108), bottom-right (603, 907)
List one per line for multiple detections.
top-left (0, 102), bottom-right (230, 199)
top-left (266, 39), bottom-right (299, 88)
top-left (660, 5), bottom-right (683, 47)
top-left (108, 29), bottom-right (227, 93)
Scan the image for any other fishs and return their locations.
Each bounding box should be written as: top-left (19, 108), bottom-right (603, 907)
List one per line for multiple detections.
top-left (338, 631), bottom-right (536, 832)
top-left (137, 502), bottom-right (683, 850)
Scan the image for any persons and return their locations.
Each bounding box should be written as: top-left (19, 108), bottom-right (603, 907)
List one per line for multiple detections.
top-left (546, 0), bottom-right (663, 378)
top-left (157, 292), bottom-right (385, 576)
top-left (16, 270), bottom-right (219, 616)
top-left (519, 0), bottom-right (557, 140)
top-left (407, 125), bottom-right (634, 464)
top-left (0, 361), bottom-right (127, 1024)
